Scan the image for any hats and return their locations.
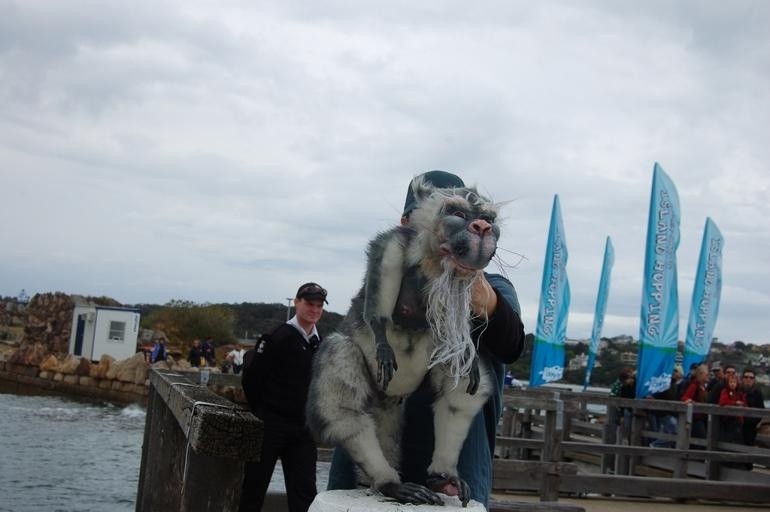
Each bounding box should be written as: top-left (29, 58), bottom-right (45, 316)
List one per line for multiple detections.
top-left (403, 171), bottom-right (466, 218)
top-left (297, 283), bottom-right (329, 305)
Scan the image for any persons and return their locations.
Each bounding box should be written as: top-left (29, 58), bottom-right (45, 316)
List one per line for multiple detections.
top-left (239, 281), bottom-right (328, 511)
top-left (225, 342), bottom-right (246, 373)
top-left (151, 337), bottom-right (168, 362)
top-left (603, 360), bottom-right (765, 471)
top-left (203, 336), bottom-right (216, 368)
top-left (316, 170), bottom-right (524, 506)
top-left (187, 339), bottom-right (202, 368)
